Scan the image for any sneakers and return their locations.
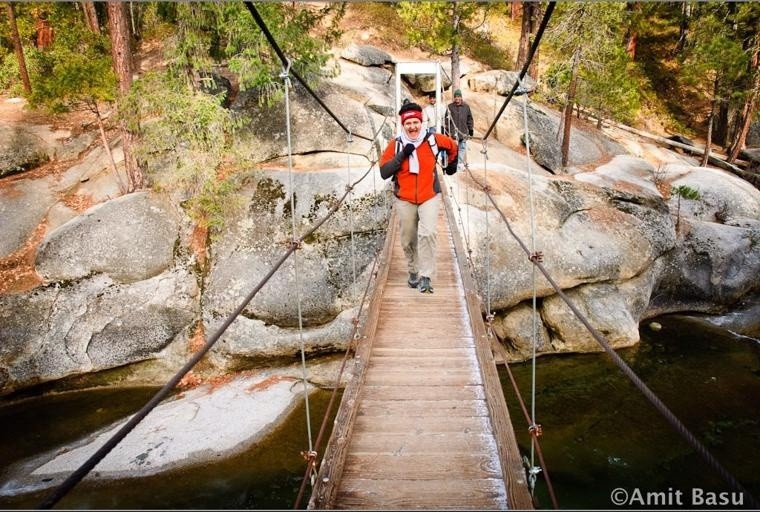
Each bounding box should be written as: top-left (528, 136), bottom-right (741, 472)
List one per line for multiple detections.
top-left (408, 272), bottom-right (419, 288)
top-left (420, 276), bottom-right (434, 294)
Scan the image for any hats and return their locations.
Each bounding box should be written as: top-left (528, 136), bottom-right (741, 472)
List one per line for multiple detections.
top-left (454, 89), bottom-right (462, 97)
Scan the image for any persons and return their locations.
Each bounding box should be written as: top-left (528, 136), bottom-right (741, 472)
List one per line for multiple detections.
top-left (444, 88), bottom-right (475, 169)
top-left (376, 102), bottom-right (460, 296)
top-left (401, 98), bottom-right (410, 105)
top-left (421, 92), bottom-right (446, 168)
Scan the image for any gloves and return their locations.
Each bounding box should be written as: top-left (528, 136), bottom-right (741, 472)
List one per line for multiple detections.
top-left (446, 163), bottom-right (457, 175)
top-left (469, 130), bottom-right (473, 136)
top-left (403, 144), bottom-right (415, 158)
